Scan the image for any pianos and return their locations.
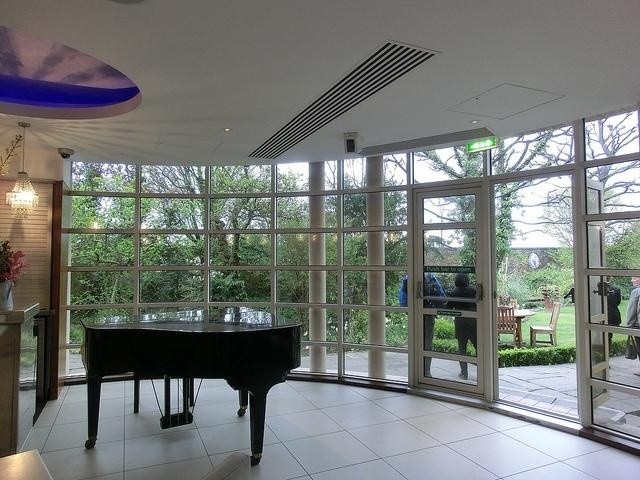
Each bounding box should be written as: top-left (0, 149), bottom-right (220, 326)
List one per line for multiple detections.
top-left (81, 307), bottom-right (301, 467)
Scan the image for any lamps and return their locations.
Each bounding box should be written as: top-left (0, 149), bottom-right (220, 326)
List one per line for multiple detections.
top-left (6, 121), bottom-right (39, 220)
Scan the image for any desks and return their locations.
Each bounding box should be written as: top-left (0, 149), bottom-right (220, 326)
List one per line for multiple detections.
top-left (497, 308), bottom-right (536, 345)
top-left (0, 450), bottom-right (55, 480)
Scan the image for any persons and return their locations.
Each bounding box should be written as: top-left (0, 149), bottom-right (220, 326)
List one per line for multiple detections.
top-left (626, 276), bottom-right (640, 377)
top-left (447, 273), bottom-right (477, 380)
top-left (399, 272), bottom-right (448, 377)
top-left (607, 276), bottom-right (621, 369)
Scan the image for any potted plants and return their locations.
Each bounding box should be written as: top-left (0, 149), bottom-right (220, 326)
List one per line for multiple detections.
top-left (0, 239), bottom-right (31, 311)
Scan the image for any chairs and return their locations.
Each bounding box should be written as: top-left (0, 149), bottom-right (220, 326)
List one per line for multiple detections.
top-left (530, 302), bottom-right (561, 346)
top-left (499, 299), bottom-right (517, 339)
top-left (497, 306), bottom-right (520, 349)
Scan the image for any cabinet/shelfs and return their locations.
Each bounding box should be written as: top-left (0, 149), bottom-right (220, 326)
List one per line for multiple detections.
top-left (0, 302), bottom-right (41, 457)
